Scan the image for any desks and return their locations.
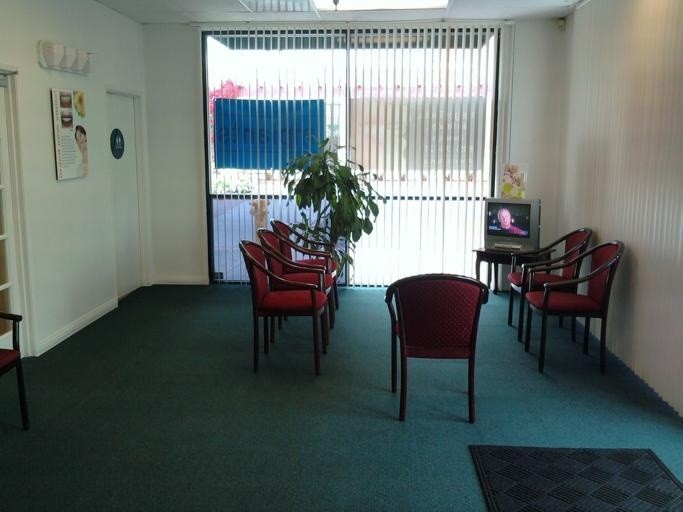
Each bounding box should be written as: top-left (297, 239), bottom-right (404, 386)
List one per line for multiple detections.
top-left (470, 247), bottom-right (554, 299)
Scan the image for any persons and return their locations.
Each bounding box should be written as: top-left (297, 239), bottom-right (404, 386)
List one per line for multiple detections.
top-left (74, 125), bottom-right (86, 174)
top-left (496, 208), bottom-right (524, 235)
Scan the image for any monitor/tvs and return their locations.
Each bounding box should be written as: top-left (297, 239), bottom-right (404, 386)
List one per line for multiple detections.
top-left (484, 198), bottom-right (541, 256)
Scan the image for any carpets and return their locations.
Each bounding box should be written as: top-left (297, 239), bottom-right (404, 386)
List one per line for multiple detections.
top-left (465, 440), bottom-right (682, 511)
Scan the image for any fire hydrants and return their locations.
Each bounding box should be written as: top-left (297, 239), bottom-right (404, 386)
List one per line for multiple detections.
top-left (248, 196), bottom-right (270, 246)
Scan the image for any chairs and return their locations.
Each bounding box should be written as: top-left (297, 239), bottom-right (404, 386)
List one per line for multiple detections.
top-left (235, 216), bottom-right (340, 375)
top-left (523, 240), bottom-right (624, 373)
top-left (0, 312), bottom-right (32, 430)
top-left (508, 226), bottom-right (592, 343)
top-left (384, 271), bottom-right (489, 424)
